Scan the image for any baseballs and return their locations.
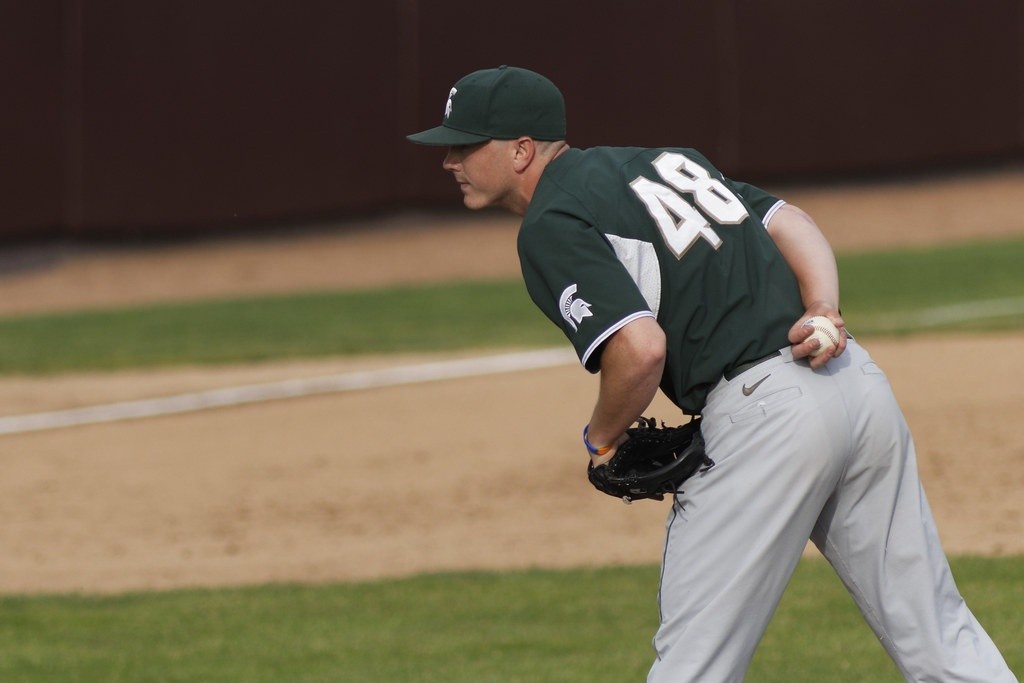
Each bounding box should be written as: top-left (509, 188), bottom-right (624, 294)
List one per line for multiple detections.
top-left (801, 315), bottom-right (840, 358)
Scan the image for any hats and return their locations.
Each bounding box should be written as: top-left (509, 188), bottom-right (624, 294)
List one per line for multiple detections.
top-left (407, 65), bottom-right (565, 146)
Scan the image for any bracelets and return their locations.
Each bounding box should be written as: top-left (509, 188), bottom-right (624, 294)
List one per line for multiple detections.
top-left (583, 424), bottom-right (613, 456)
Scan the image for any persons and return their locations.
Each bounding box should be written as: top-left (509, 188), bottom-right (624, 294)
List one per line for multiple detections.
top-left (441, 68), bottom-right (1024, 683)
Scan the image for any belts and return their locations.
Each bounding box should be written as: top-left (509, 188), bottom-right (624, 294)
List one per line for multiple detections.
top-left (724, 328), bottom-right (851, 381)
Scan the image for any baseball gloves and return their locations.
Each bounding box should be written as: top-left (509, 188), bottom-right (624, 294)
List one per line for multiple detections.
top-left (582, 419), bottom-right (707, 500)
